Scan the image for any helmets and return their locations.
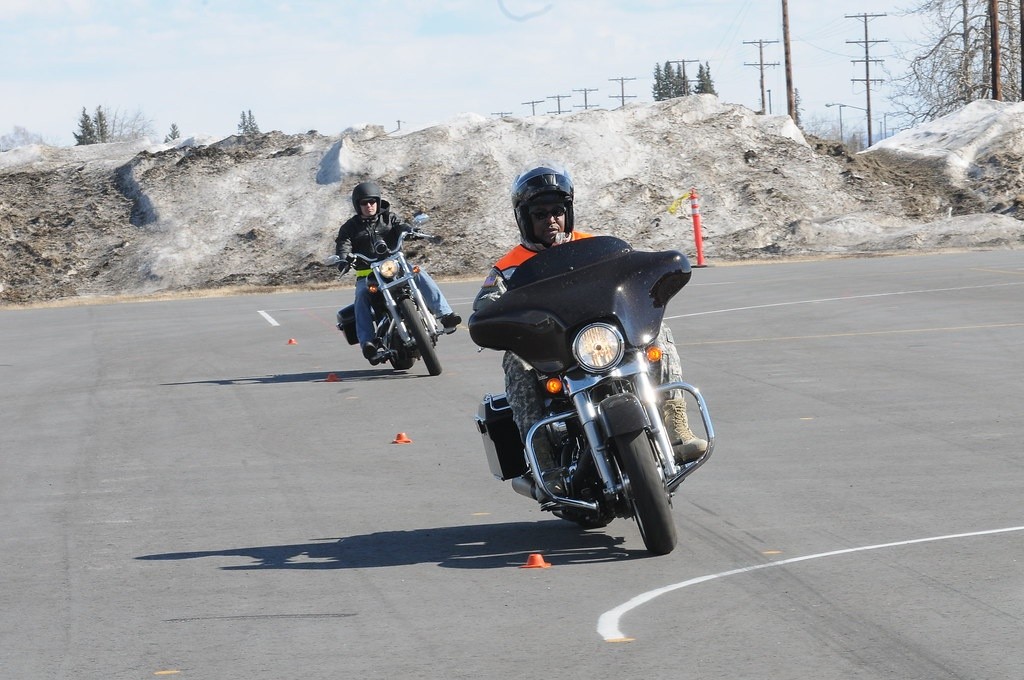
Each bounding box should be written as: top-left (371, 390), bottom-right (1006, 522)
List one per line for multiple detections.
top-left (351, 183), bottom-right (381, 217)
top-left (512, 160), bottom-right (575, 242)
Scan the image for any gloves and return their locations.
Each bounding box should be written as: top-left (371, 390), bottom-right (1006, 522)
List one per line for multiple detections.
top-left (338, 261), bottom-right (352, 272)
top-left (405, 233), bottom-right (424, 240)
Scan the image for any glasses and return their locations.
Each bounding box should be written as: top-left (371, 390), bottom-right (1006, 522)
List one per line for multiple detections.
top-left (529, 206), bottom-right (568, 220)
top-left (359, 199), bottom-right (378, 206)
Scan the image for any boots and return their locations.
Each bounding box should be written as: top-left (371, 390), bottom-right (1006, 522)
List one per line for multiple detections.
top-left (657, 398), bottom-right (708, 462)
top-left (532, 437), bottom-right (564, 504)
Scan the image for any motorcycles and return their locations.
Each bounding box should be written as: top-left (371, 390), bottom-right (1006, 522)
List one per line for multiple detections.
top-left (326, 214), bottom-right (457, 375)
top-left (468, 235), bottom-right (716, 555)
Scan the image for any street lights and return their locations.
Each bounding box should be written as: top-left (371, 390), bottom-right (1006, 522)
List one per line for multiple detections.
top-left (825, 104), bottom-right (842, 142)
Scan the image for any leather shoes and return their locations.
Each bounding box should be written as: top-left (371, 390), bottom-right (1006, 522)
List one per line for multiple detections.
top-left (441, 311), bottom-right (462, 335)
top-left (362, 340), bottom-right (381, 366)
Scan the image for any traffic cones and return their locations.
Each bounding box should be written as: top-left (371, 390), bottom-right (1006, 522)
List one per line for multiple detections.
top-left (392, 433), bottom-right (412, 444)
top-left (287, 339), bottom-right (296, 345)
top-left (325, 373), bottom-right (342, 383)
top-left (518, 553), bottom-right (553, 568)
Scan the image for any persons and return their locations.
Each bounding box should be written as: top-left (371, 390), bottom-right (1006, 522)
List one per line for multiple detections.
top-left (472, 165), bottom-right (708, 503)
top-left (336, 181), bottom-right (462, 359)
